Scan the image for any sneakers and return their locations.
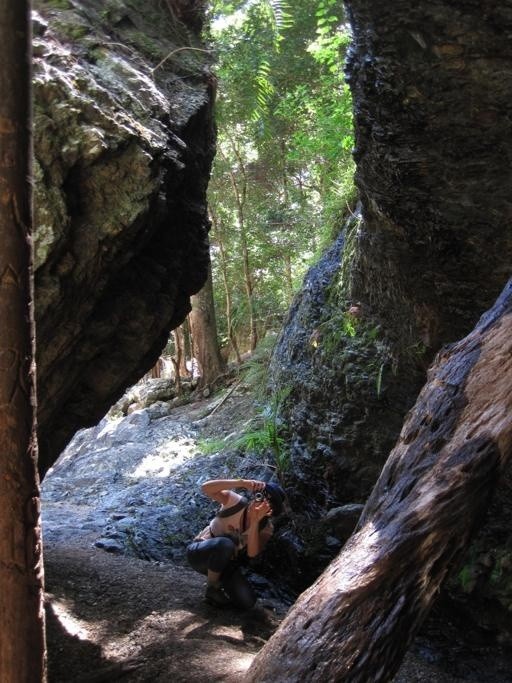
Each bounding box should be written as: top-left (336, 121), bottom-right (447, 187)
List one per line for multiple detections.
top-left (206, 584), bottom-right (241, 613)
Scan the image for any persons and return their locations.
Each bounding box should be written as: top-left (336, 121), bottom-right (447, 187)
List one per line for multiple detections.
top-left (185, 478), bottom-right (287, 615)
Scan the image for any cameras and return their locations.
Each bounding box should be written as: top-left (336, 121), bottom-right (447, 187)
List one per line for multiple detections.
top-left (253, 490), bottom-right (268, 502)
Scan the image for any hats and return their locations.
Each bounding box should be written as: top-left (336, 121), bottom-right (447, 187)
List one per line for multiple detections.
top-left (265, 483), bottom-right (285, 514)
